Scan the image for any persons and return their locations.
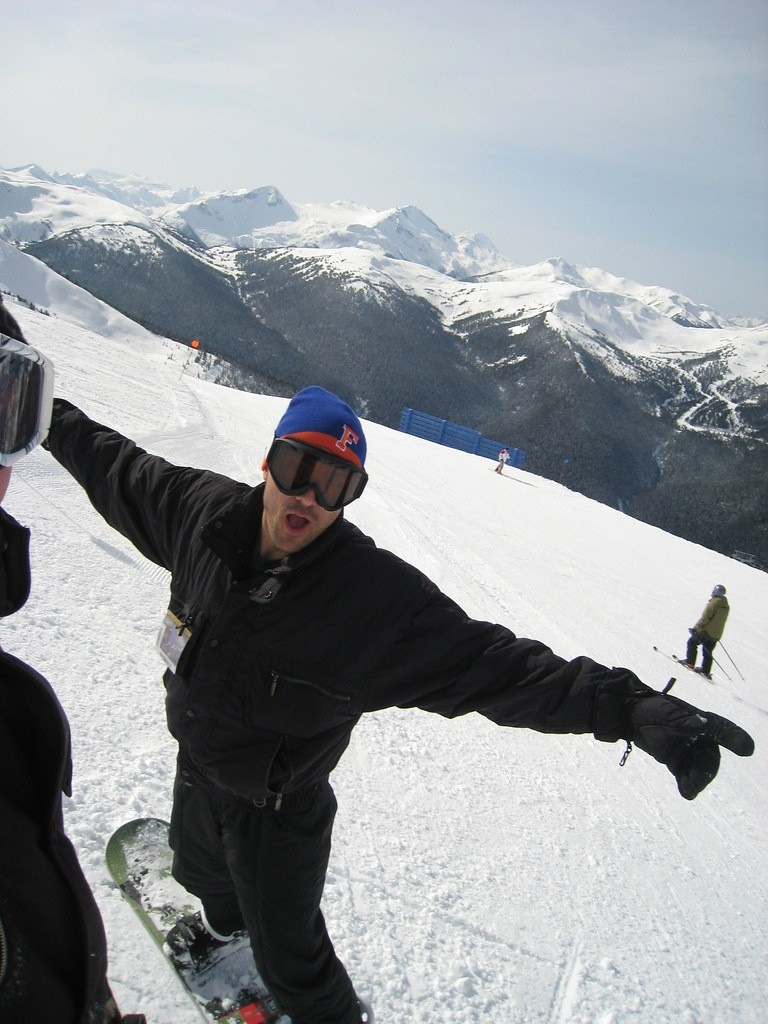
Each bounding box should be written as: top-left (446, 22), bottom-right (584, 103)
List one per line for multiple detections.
top-left (0, 299), bottom-right (754, 1024)
top-left (678, 584), bottom-right (730, 678)
top-left (0, 327), bottom-right (133, 1024)
top-left (493, 448), bottom-right (510, 474)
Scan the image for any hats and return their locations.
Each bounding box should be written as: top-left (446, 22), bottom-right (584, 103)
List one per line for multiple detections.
top-left (274, 385), bottom-right (367, 468)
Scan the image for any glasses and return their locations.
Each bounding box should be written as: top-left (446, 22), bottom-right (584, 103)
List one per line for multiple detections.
top-left (0, 338), bottom-right (54, 466)
top-left (267, 439), bottom-right (369, 509)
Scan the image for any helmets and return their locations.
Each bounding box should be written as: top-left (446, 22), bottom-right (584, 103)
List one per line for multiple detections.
top-left (714, 584), bottom-right (726, 597)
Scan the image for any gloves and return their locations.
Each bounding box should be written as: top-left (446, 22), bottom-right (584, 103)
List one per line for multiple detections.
top-left (689, 627), bottom-right (695, 633)
top-left (591, 666), bottom-right (754, 801)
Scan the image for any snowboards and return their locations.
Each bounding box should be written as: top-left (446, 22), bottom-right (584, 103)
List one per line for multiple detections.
top-left (105, 817), bottom-right (292, 1024)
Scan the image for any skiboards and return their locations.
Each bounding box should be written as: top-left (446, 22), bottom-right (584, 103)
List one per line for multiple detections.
top-left (653, 645), bottom-right (717, 684)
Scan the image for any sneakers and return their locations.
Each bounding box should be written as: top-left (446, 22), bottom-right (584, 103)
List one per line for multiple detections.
top-left (678, 659), bottom-right (694, 670)
top-left (262, 998), bottom-right (375, 1024)
top-left (694, 667), bottom-right (709, 676)
top-left (164, 910), bottom-right (247, 975)
